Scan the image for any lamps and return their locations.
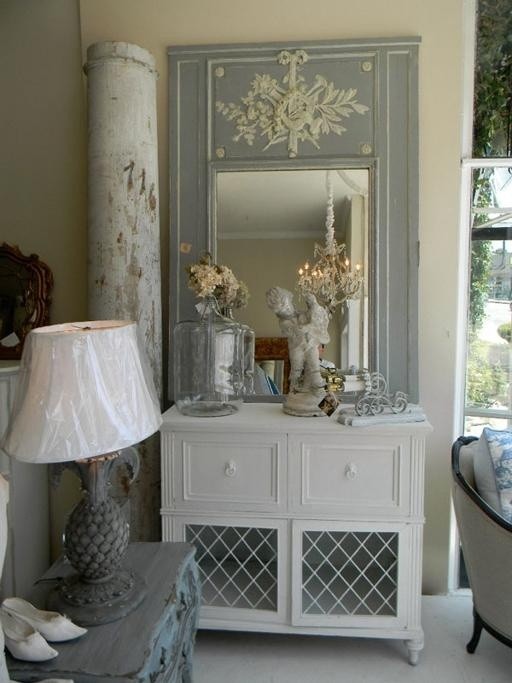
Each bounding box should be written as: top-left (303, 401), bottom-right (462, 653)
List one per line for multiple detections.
top-left (3, 318), bottom-right (167, 624)
top-left (296, 165), bottom-right (366, 306)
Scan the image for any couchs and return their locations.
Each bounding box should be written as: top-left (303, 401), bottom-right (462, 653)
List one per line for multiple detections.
top-left (450, 429), bottom-right (512, 657)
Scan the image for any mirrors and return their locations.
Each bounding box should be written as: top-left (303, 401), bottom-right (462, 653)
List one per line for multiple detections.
top-left (167, 38), bottom-right (416, 401)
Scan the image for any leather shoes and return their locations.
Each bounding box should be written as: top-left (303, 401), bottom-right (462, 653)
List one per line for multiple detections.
top-left (1, 593), bottom-right (88, 645)
top-left (2, 608), bottom-right (59, 664)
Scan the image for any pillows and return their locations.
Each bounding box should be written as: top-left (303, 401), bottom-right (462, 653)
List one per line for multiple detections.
top-left (474, 429), bottom-right (511, 516)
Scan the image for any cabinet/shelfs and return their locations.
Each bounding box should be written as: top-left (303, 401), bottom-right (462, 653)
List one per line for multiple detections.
top-left (7, 540), bottom-right (199, 681)
top-left (158, 396), bottom-right (431, 659)
